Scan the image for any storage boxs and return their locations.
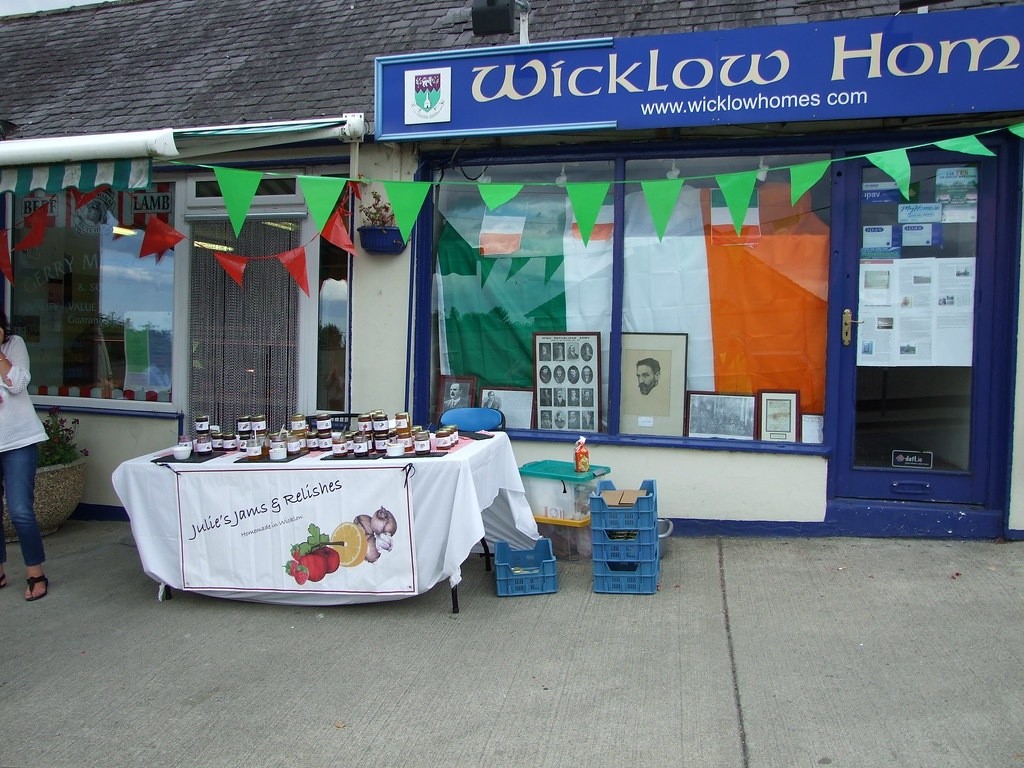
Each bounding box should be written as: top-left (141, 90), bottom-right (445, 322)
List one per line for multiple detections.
top-left (494, 459), bottom-right (661, 597)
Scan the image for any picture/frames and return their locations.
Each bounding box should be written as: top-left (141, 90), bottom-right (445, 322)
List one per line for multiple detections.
top-left (683, 390), bottom-right (758, 443)
top-left (761, 390), bottom-right (801, 442)
top-left (436, 375), bottom-right (476, 428)
top-left (481, 332), bottom-right (603, 434)
top-left (618, 332), bottom-right (688, 437)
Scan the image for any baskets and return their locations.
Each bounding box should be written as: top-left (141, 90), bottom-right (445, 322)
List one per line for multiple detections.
top-left (495, 538), bottom-right (558, 596)
top-left (589, 479), bottom-right (660, 594)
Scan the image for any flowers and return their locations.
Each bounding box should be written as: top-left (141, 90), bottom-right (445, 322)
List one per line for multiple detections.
top-left (35, 407), bottom-right (90, 464)
top-left (358, 191), bottom-right (395, 227)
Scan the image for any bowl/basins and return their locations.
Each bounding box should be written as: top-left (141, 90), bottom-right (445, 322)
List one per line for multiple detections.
top-left (172, 447), bottom-right (191, 459)
top-left (387, 444), bottom-right (405, 457)
top-left (269, 448), bottom-right (287, 460)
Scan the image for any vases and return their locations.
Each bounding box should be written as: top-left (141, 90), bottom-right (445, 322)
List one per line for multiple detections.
top-left (6, 456), bottom-right (89, 539)
top-left (357, 226), bottom-right (407, 255)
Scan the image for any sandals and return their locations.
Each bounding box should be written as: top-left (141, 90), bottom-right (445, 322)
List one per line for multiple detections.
top-left (26, 574), bottom-right (48, 601)
top-left (0, 574), bottom-right (6, 588)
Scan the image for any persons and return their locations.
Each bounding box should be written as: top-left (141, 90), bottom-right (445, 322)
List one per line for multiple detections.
top-left (0, 307), bottom-right (49, 601)
top-left (483, 391), bottom-right (499, 410)
top-left (540, 390), bottom-right (593, 407)
top-left (541, 411), bottom-right (594, 430)
top-left (539, 343), bottom-right (592, 361)
top-left (443, 383), bottom-right (468, 412)
top-left (540, 366), bottom-right (592, 384)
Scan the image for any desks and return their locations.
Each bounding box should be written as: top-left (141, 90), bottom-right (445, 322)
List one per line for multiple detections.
top-left (124, 432), bottom-right (506, 611)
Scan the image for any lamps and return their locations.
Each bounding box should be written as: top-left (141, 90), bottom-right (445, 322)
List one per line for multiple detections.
top-left (555, 164), bottom-right (570, 189)
top-left (478, 166), bottom-right (491, 183)
top-left (753, 158), bottom-right (768, 188)
top-left (664, 161), bottom-right (681, 179)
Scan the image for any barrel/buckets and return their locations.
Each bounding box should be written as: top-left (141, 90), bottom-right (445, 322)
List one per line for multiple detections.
top-left (658, 519), bottom-right (673, 557)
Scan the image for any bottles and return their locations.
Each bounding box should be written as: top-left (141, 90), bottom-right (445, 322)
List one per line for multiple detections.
top-left (574, 442), bottom-right (589, 472)
top-left (513, 568), bottom-right (521, 574)
